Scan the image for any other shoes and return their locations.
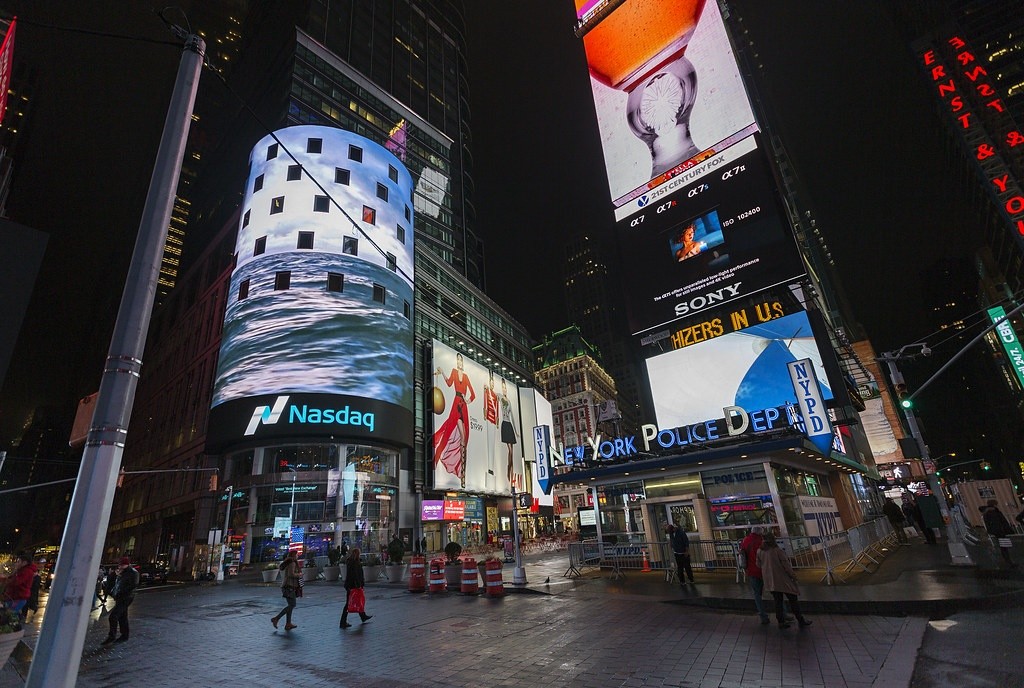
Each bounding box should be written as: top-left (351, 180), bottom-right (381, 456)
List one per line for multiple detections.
top-left (784, 615), bottom-right (794, 621)
top-left (779, 624), bottom-right (791, 630)
top-left (101, 637), bottom-right (116, 645)
top-left (899, 542), bottom-right (903, 545)
top-left (33, 608), bottom-right (38, 614)
top-left (687, 580), bottom-right (694, 584)
top-left (340, 624), bottom-right (352, 628)
top-left (362, 616), bottom-right (373, 622)
top-left (271, 617), bottom-right (278, 629)
top-left (904, 542), bottom-right (912, 545)
top-left (800, 620), bottom-right (813, 625)
top-left (681, 582), bottom-right (686, 586)
top-left (761, 619), bottom-right (770, 624)
top-left (114, 635), bottom-right (129, 643)
top-left (285, 623), bottom-right (297, 630)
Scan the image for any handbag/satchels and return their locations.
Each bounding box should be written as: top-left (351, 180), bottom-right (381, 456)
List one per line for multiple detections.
top-left (348, 585), bottom-right (366, 613)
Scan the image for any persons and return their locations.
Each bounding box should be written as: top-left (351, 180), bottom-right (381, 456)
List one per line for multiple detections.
top-left (755, 531), bottom-right (813, 632)
top-left (977, 505), bottom-right (1000, 550)
top-left (339, 549), bottom-right (373, 629)
top-left (667, 523), bottom-right (695, 586)
top-left (0, 552), bottom-right (38, 616)
top-left (875, 485), bottom-right (945, 550)
top-left (982, 499), bottom-right (1019, 569)
top-left (737, 525), bottom-right (772, 626)
top-left (99, 556), bottom-right (139, 645)
top-left (270, 549), bottom-right (305, 631)
top-left (432, 352), bottom-right (477, 489)
top-left (88, 565), bottom-right (118, 612)
top-left (17, 568), bottom-right (41, 627)
top-left (482, 377), bottom-right (500, 474)
top-left (315, 532), bottom-right (433, 569)
top-left (495, 377), bottom-right (521, 482)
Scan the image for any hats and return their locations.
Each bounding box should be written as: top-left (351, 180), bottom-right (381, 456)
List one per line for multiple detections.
top-left (119, 557), bottom-right (129, 565)
